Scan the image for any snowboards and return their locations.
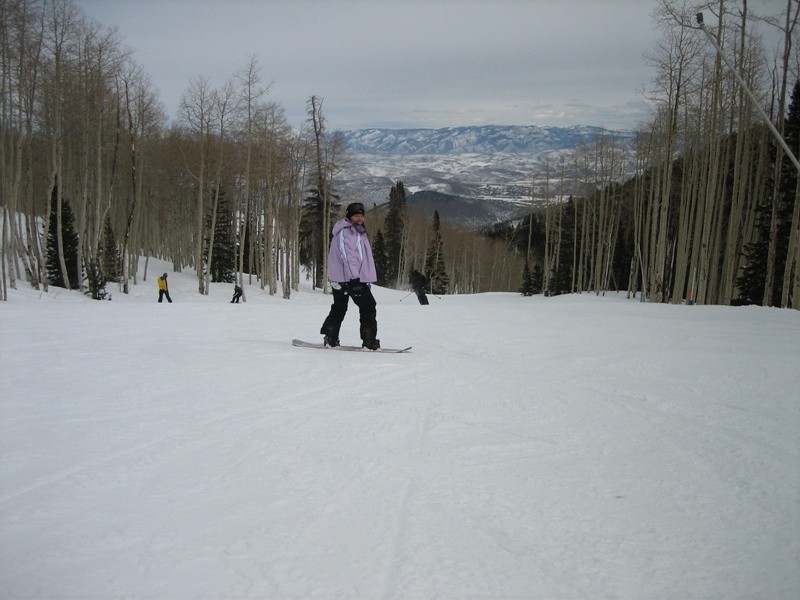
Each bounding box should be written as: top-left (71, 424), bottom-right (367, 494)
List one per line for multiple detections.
top-left (292, 339), bottom-right (412, 353)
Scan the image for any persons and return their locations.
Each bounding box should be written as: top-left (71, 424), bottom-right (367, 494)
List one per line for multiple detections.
top-left (157, 273), bottom-right (172, 303)
top-left (409, 268), bottom-right (429, 306)
top-left (230, 285), bottom-right (242, 303)
top-left (320, 202), bottom-right (380, 352)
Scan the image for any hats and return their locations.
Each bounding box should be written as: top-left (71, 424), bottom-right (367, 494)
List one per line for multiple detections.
top-left (345, 203), bottom-right (364, 219)
top-left (163, 273), bottom-right (167, 277)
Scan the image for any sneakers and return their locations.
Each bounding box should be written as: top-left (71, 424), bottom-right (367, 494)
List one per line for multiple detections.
top-left (323, 336), bottom-right (340, 347)
top-left (362, 338), bottom-right (380, 350)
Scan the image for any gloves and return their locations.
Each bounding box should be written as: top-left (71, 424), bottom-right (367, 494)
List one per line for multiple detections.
top-left (348, 278), bottom-right (363, 296)
